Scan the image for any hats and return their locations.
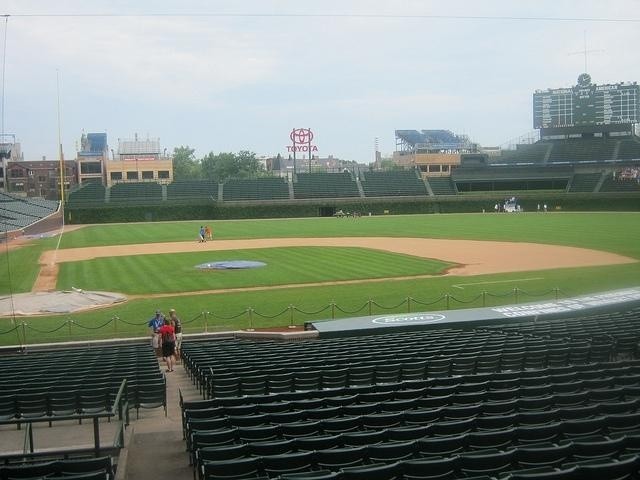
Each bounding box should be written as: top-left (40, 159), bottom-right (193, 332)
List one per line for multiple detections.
top-left (155, 309), bottom-right (161, 315)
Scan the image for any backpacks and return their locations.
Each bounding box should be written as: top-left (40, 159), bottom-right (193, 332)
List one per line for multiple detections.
top-left (164, 334), bottom-right (175, 351)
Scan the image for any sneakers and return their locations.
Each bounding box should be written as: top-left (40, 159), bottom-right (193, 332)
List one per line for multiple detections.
top-left (165, 369), bottom-right (174, 373)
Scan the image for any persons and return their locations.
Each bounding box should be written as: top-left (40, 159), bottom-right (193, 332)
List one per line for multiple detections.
top-left (148, 308), bottom-right (182, 372)
top-left (199, 225), bottom-right (212, 243)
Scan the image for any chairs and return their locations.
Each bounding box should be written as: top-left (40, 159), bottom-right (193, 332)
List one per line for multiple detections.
top-left (0, 308), bottom-right (640, 480)
